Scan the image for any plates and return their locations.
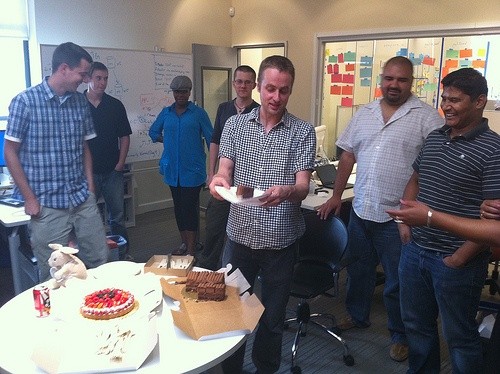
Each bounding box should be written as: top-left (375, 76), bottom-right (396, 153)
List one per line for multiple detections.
top-left (94, 261), bottom-right (141, 279)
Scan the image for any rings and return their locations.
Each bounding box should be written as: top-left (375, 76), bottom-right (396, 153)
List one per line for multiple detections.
top-left (481, 211), bottom-right (485, 216)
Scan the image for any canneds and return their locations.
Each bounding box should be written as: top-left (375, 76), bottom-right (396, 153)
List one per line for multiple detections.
top-left (33, 286), bottom-right (51, 318)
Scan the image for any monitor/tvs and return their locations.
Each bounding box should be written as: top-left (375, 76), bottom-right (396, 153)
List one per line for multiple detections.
top-left (0, 130), bottom-right (8, 167)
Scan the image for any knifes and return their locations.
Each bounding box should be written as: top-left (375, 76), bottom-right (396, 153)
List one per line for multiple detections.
top-left (167, 253), bottom-right (171, 268)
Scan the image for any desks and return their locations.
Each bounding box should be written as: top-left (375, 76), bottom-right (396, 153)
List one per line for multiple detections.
top-left (0, 263), bottom-right (248, 373)
top-left (301, 159), bottom-right (358, 212)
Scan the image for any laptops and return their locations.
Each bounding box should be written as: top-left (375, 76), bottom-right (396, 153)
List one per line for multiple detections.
top-left (315, 164), bottom-right (355, 189)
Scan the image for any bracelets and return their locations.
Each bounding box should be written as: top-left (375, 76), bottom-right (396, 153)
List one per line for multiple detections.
top-left (428, 208), bottom-right (434, 227)
top-left (395, 220), bottom-right (404, 223)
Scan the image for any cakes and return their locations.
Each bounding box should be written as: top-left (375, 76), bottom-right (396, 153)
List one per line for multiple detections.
top-left (80, 288), bottom-right (135, 320)
top-left (185, 271), bottom-right (224, 292)
top-left (236, 185), bottom-right (254, 198)
top-left (198, 283), bottom-right (226, 302)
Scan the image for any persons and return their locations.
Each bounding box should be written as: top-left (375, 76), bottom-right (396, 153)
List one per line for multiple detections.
top-left (385, 199), bottom-right (500, 247)
top-left (200, 65), bottom-right (261, 271)
top-left (4, 42), bottom-right (109, 283)
top-left (315, 56), bottom-right (445, 361)
top-left (209, 55), bottom-right (317, 374)
top-left (148, 75), bottom-right (213, 266)
top-left (398, 68), bottom-right (500, 374)
top-left (83, 61), bottom-right (132, 260)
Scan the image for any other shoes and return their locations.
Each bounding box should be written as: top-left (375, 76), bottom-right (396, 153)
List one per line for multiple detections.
top-left (184, 252), bottom-right (198, 266)
top-left (334, 312), bottom-right (355, 330)
top-left (388, 343), bottom-right (408, 360)
top-left (173, 242), bottom-right (187, 255)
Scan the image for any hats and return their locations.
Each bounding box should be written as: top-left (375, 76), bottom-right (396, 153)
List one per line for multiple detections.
top-left (170, 76), bottom-right (192, 91)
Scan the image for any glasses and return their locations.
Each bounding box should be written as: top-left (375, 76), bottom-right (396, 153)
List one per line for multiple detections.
top-left (234, 79), bottom-right (252, 86)
top-left (175, 91), bottom-right (187, 94)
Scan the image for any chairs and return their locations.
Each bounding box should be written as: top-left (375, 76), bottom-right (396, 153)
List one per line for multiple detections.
top-left (281, 212), bottom-right (356, 373)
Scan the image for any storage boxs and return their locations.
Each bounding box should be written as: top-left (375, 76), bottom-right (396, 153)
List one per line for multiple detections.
top-left (143, 255), bottom-right (195, 277)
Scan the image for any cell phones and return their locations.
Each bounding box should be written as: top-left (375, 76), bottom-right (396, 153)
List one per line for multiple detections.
top-left (0, 198), bottom-right (24, 208)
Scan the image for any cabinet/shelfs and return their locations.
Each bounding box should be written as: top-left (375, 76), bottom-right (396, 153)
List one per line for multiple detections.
top-left (97, 171), bottom-right (135, 234)
